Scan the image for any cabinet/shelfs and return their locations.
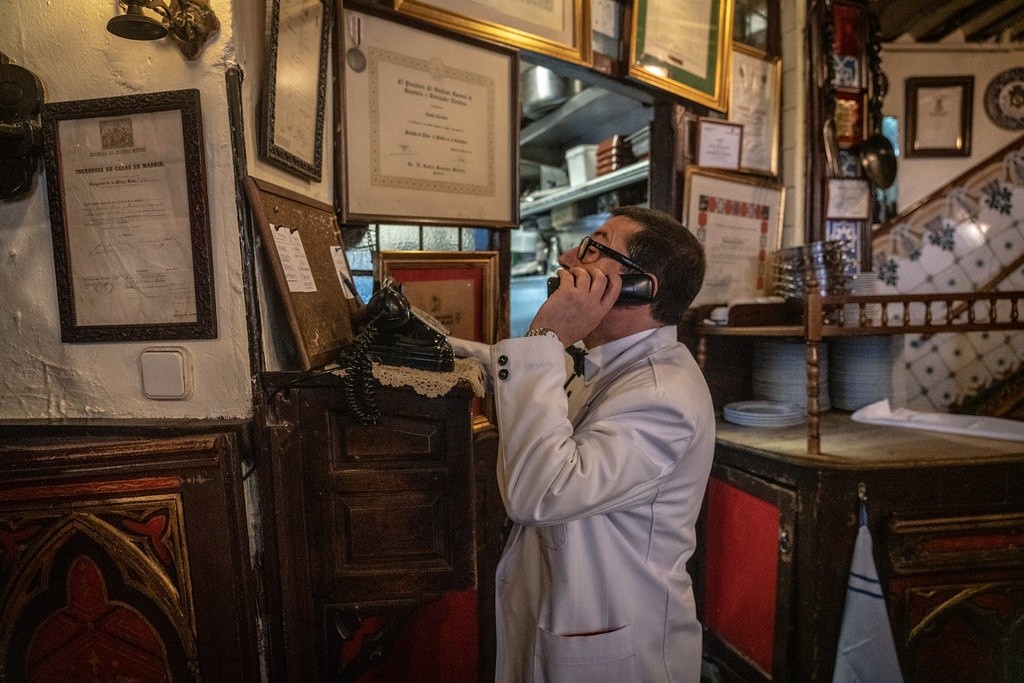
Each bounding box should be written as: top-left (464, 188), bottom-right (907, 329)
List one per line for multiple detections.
top-left (690, 280), bottom-right (1024, 683)
top-left (261, 355), bottom-right (479, 682)
top-left (510, 86), bottom-right (651, 283)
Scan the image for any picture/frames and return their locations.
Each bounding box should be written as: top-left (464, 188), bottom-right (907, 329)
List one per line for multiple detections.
top-left (904, 76), bottom-right (974, 157)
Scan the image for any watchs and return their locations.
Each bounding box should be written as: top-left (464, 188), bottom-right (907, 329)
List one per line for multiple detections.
top-left (525, 328), bottom-right (561, 341)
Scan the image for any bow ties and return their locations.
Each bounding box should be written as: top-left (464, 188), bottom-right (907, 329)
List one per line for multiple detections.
top-left (565, 344), bottom-right (589, 377)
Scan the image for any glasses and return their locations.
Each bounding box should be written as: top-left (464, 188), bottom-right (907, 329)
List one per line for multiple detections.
top-left (577, 236), bottom-right (645, 275)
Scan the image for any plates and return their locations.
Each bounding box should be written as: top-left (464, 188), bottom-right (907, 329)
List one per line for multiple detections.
top-left (723, 402), bottom-right (808, 428)
top-left (749, 337), bottom-right (831, 414)
top-left (831, 331), bottom-right (908, 411)
top-left (842, 274), bottom-right (886, 329)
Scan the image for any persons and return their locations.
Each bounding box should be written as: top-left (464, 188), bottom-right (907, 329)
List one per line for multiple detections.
top-left (489, 205), bottom-right (716, 683)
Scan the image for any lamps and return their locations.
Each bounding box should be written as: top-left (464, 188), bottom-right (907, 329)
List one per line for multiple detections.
top-left (106, 0), bottom-right (219, 61)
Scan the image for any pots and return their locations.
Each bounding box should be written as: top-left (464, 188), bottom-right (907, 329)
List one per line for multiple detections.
top-left (519, 64), bottom-right (569, 120)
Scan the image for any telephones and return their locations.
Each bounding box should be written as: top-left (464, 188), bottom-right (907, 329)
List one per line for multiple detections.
top-left (547, 274), bottom-right (655, 305)
top-left (354, 276), bottom-right (456, 372)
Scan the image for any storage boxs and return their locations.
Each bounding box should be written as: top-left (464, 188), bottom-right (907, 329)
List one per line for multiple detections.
top-left (564, 144), bottom-right (599, 188)
